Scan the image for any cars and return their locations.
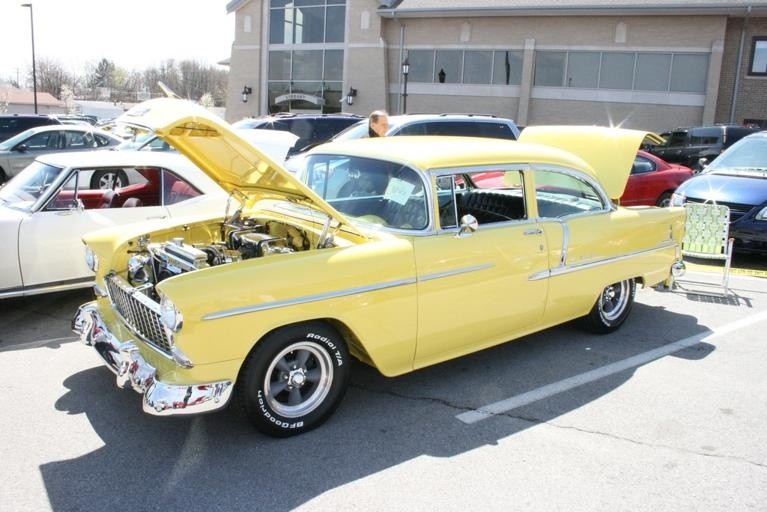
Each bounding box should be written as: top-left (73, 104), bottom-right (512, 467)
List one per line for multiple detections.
top-left (72, 111), bottom-right (689, 439)
top-left (672, 130), bottom-right (767, 262)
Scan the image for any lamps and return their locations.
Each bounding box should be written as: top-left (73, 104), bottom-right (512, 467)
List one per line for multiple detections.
top-left (240, 84), bottom-right (251, 103)
top-left (347, 86), bottom-right (357, 106)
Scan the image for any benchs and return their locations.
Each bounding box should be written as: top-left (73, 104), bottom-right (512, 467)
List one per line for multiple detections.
top-left (461, 189), bottom-right (578, 221)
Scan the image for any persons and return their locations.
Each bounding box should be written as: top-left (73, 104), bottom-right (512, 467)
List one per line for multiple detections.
top-left (366, 110), bottom-right (390, 137)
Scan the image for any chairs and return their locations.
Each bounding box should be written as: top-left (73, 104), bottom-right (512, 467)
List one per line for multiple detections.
top-left (383, 187), bottom-right (426, 228)
top-left (96, 185), bottom-right (142, 209)
